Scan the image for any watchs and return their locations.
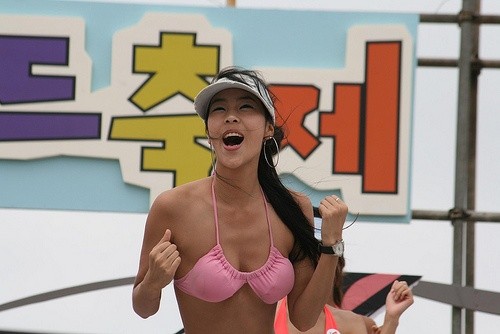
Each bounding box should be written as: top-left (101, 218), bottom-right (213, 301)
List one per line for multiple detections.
top-left (318, 238), bottom-right (344, 257)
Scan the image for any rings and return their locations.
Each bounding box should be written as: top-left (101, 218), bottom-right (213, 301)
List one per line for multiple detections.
top-left (335, 197), bottom-right (339, 201)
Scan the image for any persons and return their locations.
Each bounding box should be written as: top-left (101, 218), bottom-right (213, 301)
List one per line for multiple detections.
top-left (132, 68), bottom-right (349, 334)
top-left (273, 241), bottom-right (415, 334)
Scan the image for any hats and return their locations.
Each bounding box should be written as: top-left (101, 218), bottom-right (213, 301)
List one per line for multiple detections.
top-left (193, 74), bottom-right (277, 123)
